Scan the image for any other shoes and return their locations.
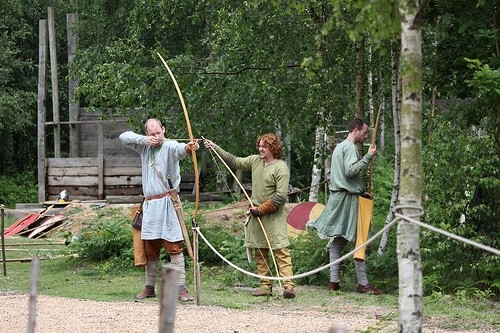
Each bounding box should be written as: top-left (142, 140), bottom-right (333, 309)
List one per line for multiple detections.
top-left (179, 289), bottom-right (193, 302)
top-left (137, 286), bottom-right (155, 298)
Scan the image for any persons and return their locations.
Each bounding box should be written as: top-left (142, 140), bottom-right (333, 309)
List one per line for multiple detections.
top-left (118, 118), bottom-right (199, 302)
top-left (305, 117), bottom-right (383, 295)
top-left (203, 133), bottom-right (296, 298)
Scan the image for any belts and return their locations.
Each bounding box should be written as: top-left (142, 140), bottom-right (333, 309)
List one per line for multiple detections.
top-left (145, 192), bottom-right (170, 200)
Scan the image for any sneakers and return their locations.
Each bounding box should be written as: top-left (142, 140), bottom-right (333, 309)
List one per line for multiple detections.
top-left (251, 287), bottom-right (272, 296)
top-left (283, 287), bottom-right (295, 298)
top-left (357, 283), bottom-right (385, 295)
top-left (328, 282), bottom-right (340, 291)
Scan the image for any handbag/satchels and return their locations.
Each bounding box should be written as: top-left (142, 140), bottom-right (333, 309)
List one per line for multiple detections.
top-left (132, 202), bottom-right (143, 228)
